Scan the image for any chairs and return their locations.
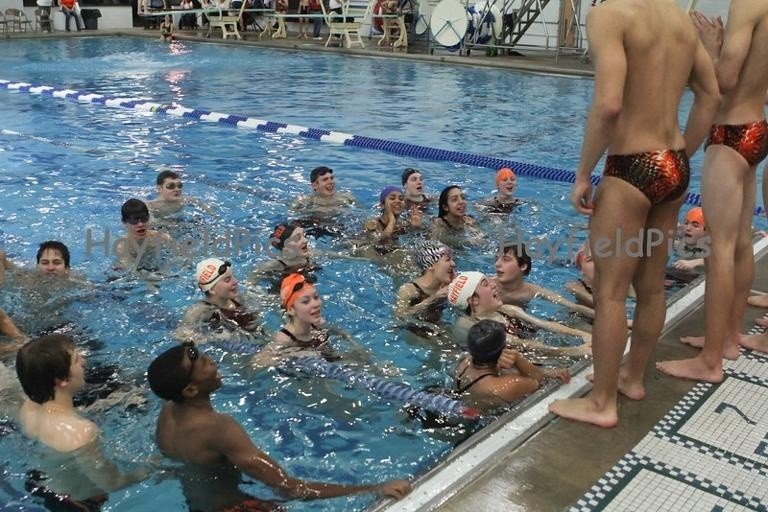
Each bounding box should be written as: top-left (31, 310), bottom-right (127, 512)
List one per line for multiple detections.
top-left (0, 8), bottom-right (56, 38)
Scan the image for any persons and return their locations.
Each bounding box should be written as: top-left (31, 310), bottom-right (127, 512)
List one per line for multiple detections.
top-left (673, 207), bottom-right (706, 271)
top-left (148, 341), bottom-right (411, 499)
top-left (548, 0), bottom-right (721, 429)
top-left (658, 0), bottom-right (768, 385)
top-left (115, 170), bottom-right (186, 251)
top-left (37, 241), bottom-right (70, 276)
top-left (185, 258), bottom-right (241, 320)
top-left (367, 166), bottom-right (595, 399)
top-left (1, 312), bottom-right (20, 335)
top-left (35, 0), bottom-right (416, 49)
top-left (297, 166), bottom-right (352, 203)
top-left (278, 273), bottom-right (326, 341)
top-left (561, 0), bottom-right (578, 50)
top-left (268, 223), bottom-right (313, 268)
top-left (17, 332), bottom-right (95, 451)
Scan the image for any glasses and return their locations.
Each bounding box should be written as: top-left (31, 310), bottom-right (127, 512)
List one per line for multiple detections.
top-left (201, 261), bottom-right (232, 285)
top-left (318, 168), bottom-right (334, 174)
top-left (182, 339), bottom-right (198, 382)
top-left (163, 181), bottom-right (184, 189)
top-left (123, 215), bottom-right (149, 224)
top-left (284, 275), bottom-right (317, 308)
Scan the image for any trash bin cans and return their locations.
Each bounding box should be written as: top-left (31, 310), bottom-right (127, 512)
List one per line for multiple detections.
top-left (81, 9), bottom-right (102, 30)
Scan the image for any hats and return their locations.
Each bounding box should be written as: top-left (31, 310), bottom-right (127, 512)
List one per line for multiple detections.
top-left (470, 320), bottom-right (506, 355)
top-left (495, 167), bottom-right (517, 182)
top-left (382, 185), bottom-right (405, 201)
top-left (196, 258), bottom-right (229, 293)
top-left (415, 240), bottom-right (450, 269)
top-left (447, 271), bottom-right (484, 310)
top-left (280, 272), bottom-right (314, 308)
top-left (685, 207), bottom-right (706, 225)
top-left (270, 222), bottom-right (296, 249)
